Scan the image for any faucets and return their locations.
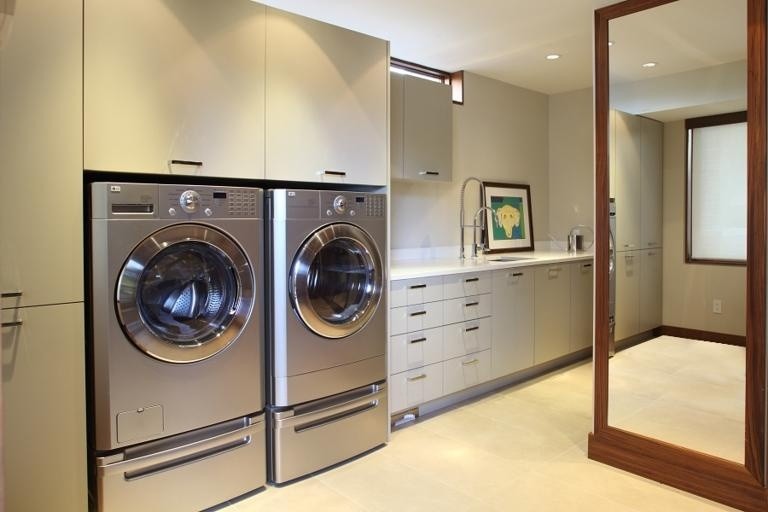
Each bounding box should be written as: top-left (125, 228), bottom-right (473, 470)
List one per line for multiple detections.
top-left (470, 206), bottom-right (496, 259)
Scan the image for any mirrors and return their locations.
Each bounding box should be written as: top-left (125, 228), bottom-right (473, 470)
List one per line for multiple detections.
top-left (590, 0), bottom-right (767, 511)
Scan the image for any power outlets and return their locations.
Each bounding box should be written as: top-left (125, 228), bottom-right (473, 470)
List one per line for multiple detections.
top-left (713, 300), bottom-right (722, 313)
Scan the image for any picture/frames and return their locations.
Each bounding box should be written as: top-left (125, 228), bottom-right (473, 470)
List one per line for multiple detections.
top-left (479, 181), bottom-right (533, 253)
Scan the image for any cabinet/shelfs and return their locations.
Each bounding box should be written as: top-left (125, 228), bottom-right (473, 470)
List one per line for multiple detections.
top-left (0, 301), bottom-right (88, 511)
top-left (390, 71), bottom-right (452, 183)
top-left (610, 110), bottom-right (661, 342)
top-left (389, 257), bottom-right (595, 415)
top-left (1, 0), bottom-right (91, 310)
top-left (83, 2), bottom-right (265, 180)
top-left (265, 5), bottom-right (388, 187)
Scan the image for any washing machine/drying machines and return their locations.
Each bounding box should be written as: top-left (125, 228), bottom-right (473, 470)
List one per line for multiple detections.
top-left (264, 186), bottom-right (389, 486)
top-left (89, 181), bottom-right (267, 512)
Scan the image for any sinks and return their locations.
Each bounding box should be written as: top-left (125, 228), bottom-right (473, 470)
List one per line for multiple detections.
top-left (488, 255), bottom-right (526, 263)
